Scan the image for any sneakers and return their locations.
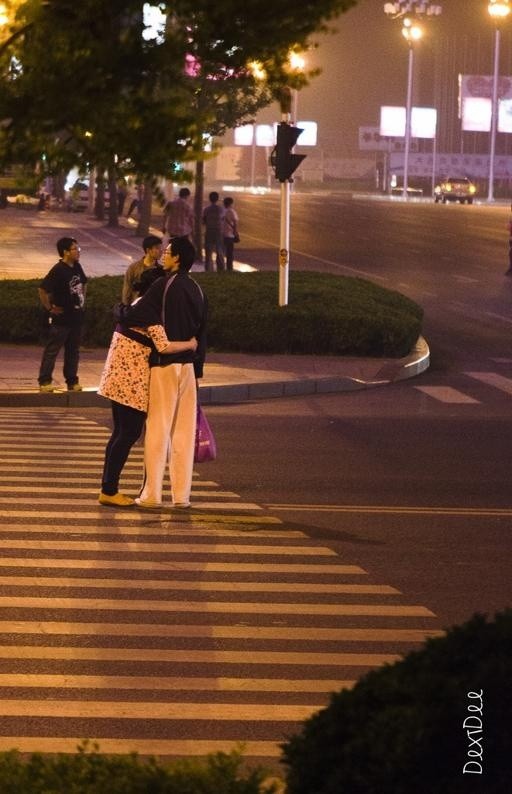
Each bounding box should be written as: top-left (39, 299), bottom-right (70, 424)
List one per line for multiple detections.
top-left (40, 384), bottom-right (52, 392)
top-left (68, 384), bottom-right (81, 391)
top-left (99, 492), bottom-right (191, 508)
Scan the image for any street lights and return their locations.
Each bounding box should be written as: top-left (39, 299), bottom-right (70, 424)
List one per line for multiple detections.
top-left (485, 0), bottom-right (511, 204)
top-left (288, 49), bottom-right (306, 193)
top-left (382, 1), bottom-right (444, 203)
top-left (244, 61), bottom-right (264, 189)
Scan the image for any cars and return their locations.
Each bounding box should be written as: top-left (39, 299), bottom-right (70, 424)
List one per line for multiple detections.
top-left (433, 175), bottom-right (476, 204)
top-left (69, 175), bottom-right (121, 212)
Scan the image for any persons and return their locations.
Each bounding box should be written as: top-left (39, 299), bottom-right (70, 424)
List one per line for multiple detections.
top-left (117, 237), bottom-right (164, 302)
top-left (112, 237), bottom-right (208, 510)
top-left (38, 238), bottom-right (88, 393)
top-left (97, 266), bottom-right (159, 511)
top-left (109, 177), bottom-right (256, 273)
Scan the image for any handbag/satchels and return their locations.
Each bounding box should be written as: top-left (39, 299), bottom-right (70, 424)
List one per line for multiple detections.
top-left (235, 233), bottom-right (239, 242)
top-left (194, 407), bottom-right (215, 463)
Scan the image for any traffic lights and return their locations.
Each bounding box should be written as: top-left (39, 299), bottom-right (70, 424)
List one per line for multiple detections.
top-left (273, 121), bottom-right (307, 184)
